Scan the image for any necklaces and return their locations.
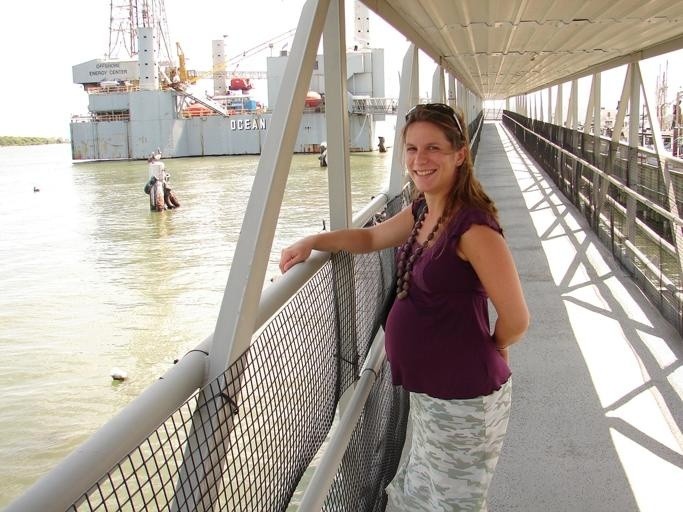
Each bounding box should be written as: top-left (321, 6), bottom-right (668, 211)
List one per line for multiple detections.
top-left (394, 200), bottom-right (450, 300)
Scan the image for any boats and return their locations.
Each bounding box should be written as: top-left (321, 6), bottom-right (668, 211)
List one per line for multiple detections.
top-left (69, 0), bottom-right (385, 160)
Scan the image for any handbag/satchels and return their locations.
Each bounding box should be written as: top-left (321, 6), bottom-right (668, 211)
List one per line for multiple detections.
top-left (378, 200), bottom-right (426, 333)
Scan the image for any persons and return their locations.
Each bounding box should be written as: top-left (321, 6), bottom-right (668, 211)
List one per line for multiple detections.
top-left (278, 99), bottom-right (531, 511)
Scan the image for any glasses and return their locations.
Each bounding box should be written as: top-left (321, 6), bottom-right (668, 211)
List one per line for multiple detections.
top-left (401, 103), bottom-right (465, 141)
